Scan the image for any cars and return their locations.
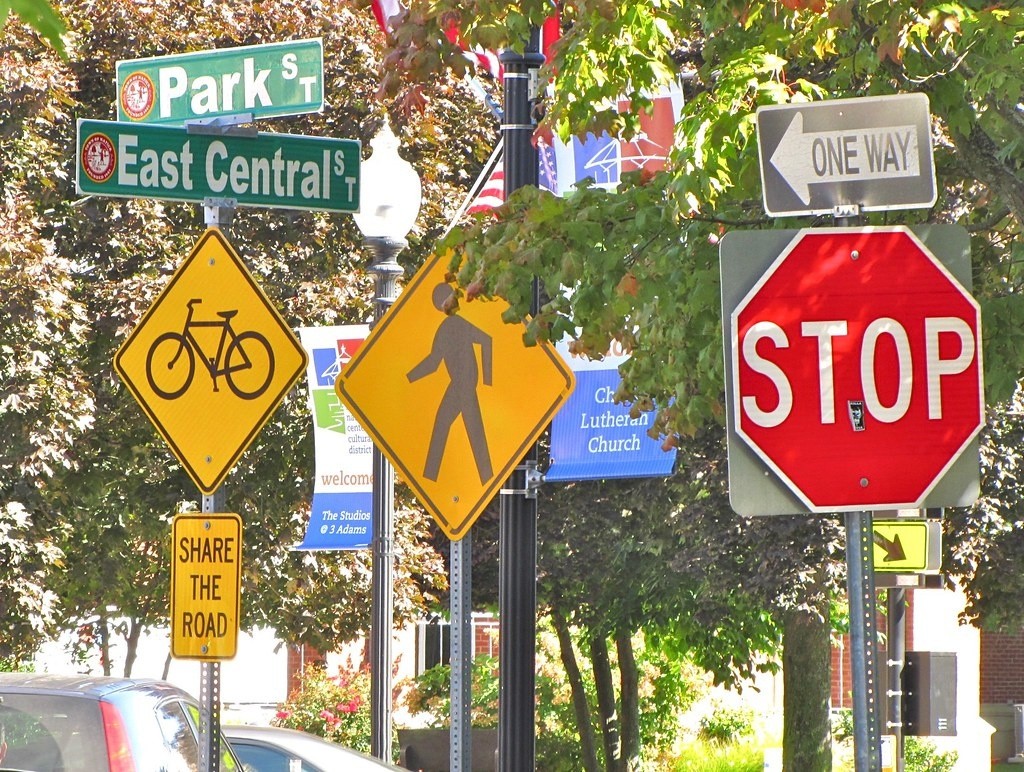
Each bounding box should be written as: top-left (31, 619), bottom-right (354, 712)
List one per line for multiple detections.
top-left (221, 724), bottom-right (414, 772)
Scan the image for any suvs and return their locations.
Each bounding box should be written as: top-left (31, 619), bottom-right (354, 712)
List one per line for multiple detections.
top-left (0, 671), bottom-right (246, 772)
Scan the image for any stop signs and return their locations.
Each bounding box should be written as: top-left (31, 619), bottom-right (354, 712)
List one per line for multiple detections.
top-left (732, 224), bottom-right (986, 509)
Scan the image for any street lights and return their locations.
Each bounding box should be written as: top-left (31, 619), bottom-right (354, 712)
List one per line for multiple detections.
top-left (349, 108), bottom-right (423, 760)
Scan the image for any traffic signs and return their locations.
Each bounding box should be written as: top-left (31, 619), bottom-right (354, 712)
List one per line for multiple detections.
top-left (753, 93), bottom-right (938, 219)
